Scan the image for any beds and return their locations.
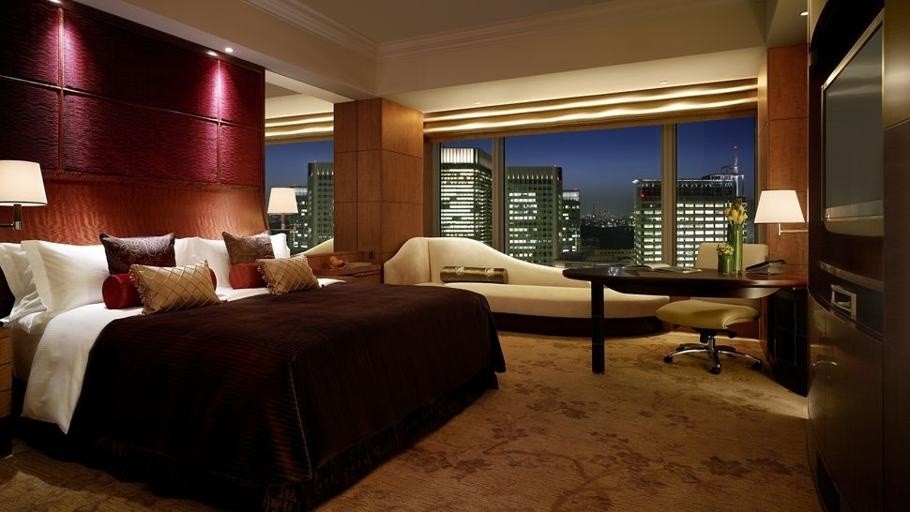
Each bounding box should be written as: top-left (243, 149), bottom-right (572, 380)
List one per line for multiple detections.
top-left (0, 233), bottom-right (506, 512)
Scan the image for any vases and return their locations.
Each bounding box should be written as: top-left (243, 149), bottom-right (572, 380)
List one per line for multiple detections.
top-left (718, 255), bottom-right (736, 274)
top-left (727, 228), bottom-right (744, 275)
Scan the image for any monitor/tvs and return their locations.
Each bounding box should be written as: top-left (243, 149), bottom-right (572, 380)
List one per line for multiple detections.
top-left (820, 9), bottom-right (883, 237)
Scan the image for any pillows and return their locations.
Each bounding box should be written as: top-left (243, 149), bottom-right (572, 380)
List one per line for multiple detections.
top-left (99, 230), bottom-right (181, 274)
top-left (103, 269), bottom-right (219, 310)
top-left (439, 265), bottom-right (509, 284)
top-left (228, 260), bottom-right (264, 288)
top-left (254, 255), bottom-right (325, 295)
top-left (128, 260), bottom-right (226, 311)
top-left (217, 229), bottom-right (276, 264)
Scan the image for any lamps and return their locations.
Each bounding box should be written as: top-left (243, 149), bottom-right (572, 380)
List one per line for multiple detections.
top-left (753, 187), bottom-right (809, 236)
top-left (266, 187), bottom-right (299, 229)
top-left (0, 160), bottom-right (49, 230)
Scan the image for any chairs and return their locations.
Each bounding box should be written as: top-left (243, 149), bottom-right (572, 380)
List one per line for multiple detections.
top-left (654, 240), bottom-right (768, 375)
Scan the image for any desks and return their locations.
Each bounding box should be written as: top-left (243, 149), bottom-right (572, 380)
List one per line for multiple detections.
top-left (561, 261), bottom-right (809, 376)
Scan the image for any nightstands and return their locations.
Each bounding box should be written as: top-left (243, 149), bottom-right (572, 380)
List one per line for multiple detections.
top-left (313, 263), bottom-right (381, 284)
top-left (0, 327), bottom-right (15, 460)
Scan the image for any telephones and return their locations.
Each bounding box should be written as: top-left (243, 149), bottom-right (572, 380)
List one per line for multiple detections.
top-left (746, 259), bottom-right (786, 277)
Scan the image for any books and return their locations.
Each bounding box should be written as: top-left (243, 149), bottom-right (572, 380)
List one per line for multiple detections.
top-left (632, 262), bottom-right (704, 274)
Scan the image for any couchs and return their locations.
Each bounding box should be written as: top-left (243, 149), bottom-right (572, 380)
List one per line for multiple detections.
top-left (382, 235), bottom-right (671, 321)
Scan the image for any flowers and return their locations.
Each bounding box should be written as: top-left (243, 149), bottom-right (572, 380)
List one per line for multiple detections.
top-left (724, 195), bottom-right (748, 226)
top-left (716, 242), bottom-right (734, 255)
